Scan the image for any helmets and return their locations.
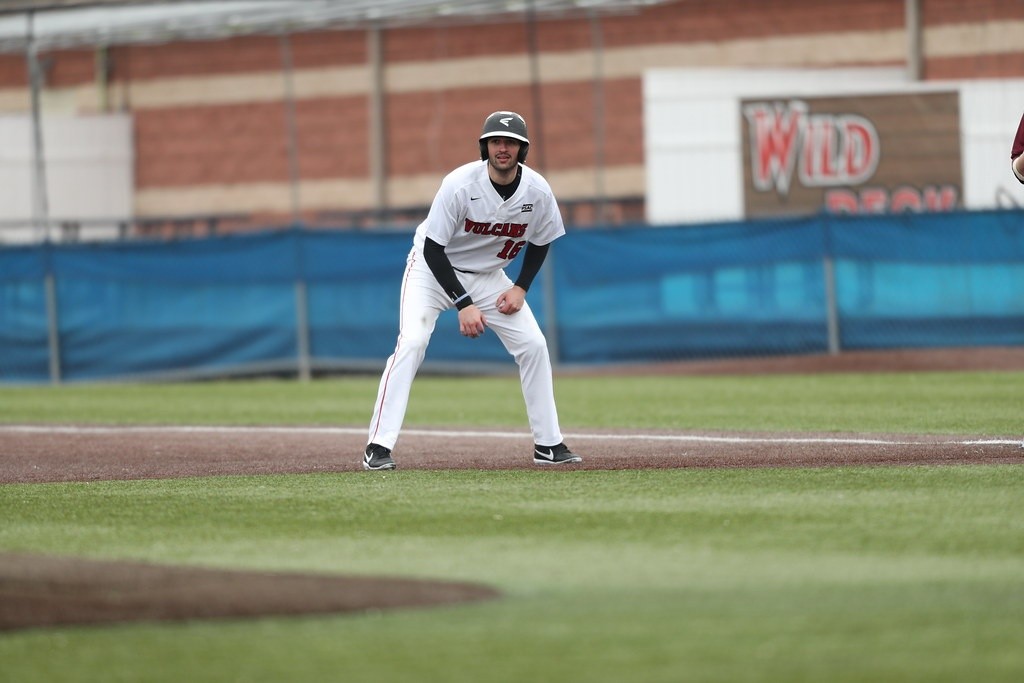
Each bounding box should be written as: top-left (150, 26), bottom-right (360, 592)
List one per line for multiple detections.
top-left (478, 110), bottom-right (530, 165)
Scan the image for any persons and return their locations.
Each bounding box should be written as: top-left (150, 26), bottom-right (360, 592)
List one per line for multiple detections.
top-left (360, 110), bottom-right (589, 471)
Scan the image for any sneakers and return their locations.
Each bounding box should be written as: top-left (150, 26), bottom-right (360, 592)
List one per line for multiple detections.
top-left (362, 442), bottom-right (396, 472)
top-left (533, 442), bottom-right (582, 465)
